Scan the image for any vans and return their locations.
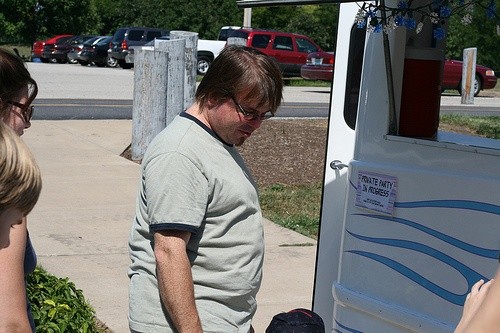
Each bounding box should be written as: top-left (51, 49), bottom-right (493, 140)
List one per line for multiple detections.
top-left (309, 2), bottom-right (500, 333)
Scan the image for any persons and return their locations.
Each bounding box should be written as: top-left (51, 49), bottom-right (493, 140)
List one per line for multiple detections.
top-left (128, 44), bottom-right (283, 333)
top-left (0, 120), bottom-right (42, 250)
top-left (266, 266), bottom-right (500, 333)
top-left (0, 48), bottom-right (37, 333)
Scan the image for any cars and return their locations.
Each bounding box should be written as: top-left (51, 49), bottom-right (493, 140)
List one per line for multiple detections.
top-left (72, 36), bottom-right (94, 65)
top-left (125, 36), bottom-right (174, 63)
top-left (298, 51), bottom-right (496, 100)
top-left (80, 36), bottom-right (118, 68)
top-left (42, 34), bottom-right (84, 62)
top-left (33, 35), bottom-right (75, 64)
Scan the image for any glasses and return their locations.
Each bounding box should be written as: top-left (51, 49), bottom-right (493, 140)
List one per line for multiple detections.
top-left (4, 98), bottom-right (34, 122)
top-left (231, 95), bottom-right (274, 119)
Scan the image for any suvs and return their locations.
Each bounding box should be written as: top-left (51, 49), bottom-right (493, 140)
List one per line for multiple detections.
top-left (225, 28), bottom-right (336, 78)
top-left (108, 28), bottom-right (165, 71)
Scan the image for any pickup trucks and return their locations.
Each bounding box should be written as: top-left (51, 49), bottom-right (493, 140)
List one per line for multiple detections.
top-left (193, 26), bottom-right (292, 76)
